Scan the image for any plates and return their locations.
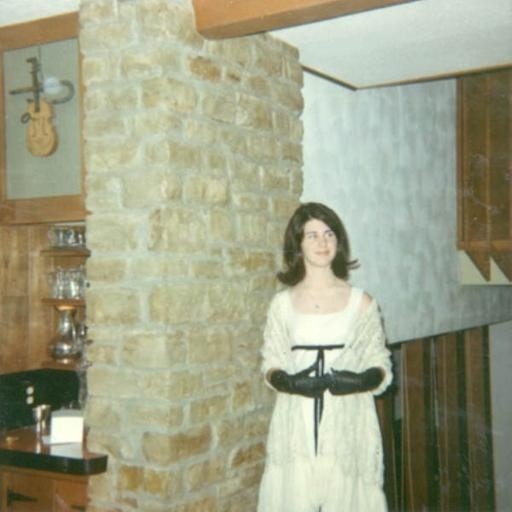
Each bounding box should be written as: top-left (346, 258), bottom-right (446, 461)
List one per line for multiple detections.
top-left (39, 222), bottom-right (88, 372)
top-left (0, 464), bottom-right (89, 512)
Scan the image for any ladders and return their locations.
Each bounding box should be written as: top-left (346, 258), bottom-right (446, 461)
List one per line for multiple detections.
top-left (8, 57), bottom-right (75, 158)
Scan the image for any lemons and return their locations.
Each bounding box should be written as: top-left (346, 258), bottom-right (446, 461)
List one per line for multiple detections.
top-left (45, 304), bottom-right (87, 364)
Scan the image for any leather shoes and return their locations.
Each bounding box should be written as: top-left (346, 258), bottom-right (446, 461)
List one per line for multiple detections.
top-left (330, 368), bottom-right (383, 394)
top-left (271, 367), bottom-right (330, 397)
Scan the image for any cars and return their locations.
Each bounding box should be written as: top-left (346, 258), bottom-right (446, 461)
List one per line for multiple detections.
top-left (43, 227), bottom-right (84, 299)
top-left (31, 402), bottom-right (50, 433)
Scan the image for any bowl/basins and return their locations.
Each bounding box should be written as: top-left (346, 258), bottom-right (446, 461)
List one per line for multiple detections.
top-left (299, 275), bottom-right (338, 311)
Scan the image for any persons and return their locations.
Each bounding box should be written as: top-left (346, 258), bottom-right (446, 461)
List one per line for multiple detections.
top-left (254, 203), bottom-right (393, 512)
